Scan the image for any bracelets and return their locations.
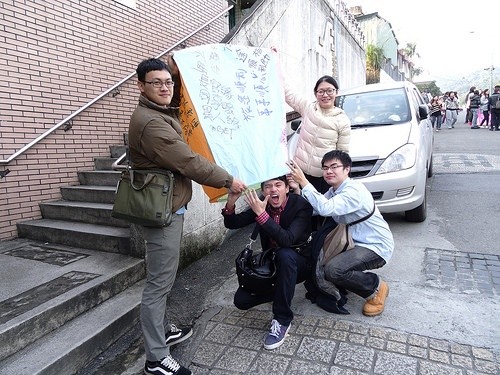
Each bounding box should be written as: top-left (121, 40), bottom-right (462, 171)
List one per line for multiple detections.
top-left (225, 202), bottom-right (236, 211)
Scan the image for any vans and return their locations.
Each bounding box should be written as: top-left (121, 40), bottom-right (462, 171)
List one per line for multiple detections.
top-left (336, 80), bottom-right (435, 223)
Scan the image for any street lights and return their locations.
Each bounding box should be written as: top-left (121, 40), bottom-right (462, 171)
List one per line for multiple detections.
top-left (469, 30), bottom-right (495, 131)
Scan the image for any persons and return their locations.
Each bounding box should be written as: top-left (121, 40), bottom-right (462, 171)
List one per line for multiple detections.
top-left (420, 85), bottom-right (500, 131)
top-left (129, 50), bottom-right (249, 375)
top-left (285, 150), bottom-right (396, 317)
top-left (221, 175), bottom-right (314, 349)
top-left (271, 45), bottom-right (350, 248)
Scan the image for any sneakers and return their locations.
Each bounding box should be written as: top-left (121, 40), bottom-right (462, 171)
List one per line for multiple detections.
top-left (166, 324), bottom-right (194, 346)
top-left (143, 354), bottom-right (194, 375)
top-left (264, 319), bottom-right (292, 350)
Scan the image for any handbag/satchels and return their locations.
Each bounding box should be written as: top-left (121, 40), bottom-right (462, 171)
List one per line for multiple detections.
top-left (112, 164), bottom-right (174, 228)
top-left (318, 223), bottom-right (355, 266)
top-left (233, 246), bottom-right (276, 298)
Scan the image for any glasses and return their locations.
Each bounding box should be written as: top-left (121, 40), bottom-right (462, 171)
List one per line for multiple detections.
top-left (316, 88), bottom-right (336, 95)
top-left (321, 165), bottom-right (343, 171)
top-left (144, 81), bottom-right (174, 88)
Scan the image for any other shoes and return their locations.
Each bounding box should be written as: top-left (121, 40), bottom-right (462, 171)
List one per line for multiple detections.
top-left (362, 281), bottom-right (390, 317)
